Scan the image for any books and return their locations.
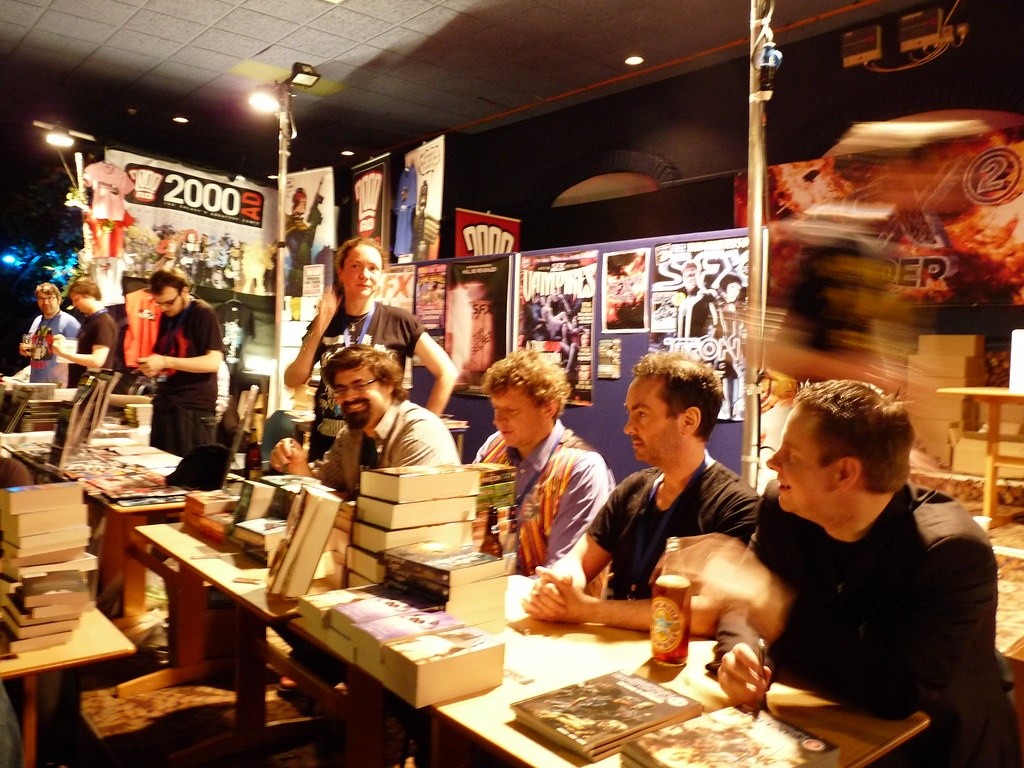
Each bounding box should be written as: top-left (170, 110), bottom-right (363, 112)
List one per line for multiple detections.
top-left (510, 670), bottom-right (701, 762)
top-left (617, 702), bottom-right (839, 767)
top-left (124, 403), bottom-right (153, 429)
top-left (0, 384), bottom-right (62, 433)
top-left (179, 462), bottom-right (520, 710)
top-left (0, 481), bottom-right (98, 658)
top-left (51, 370), bottom-right (121, 467)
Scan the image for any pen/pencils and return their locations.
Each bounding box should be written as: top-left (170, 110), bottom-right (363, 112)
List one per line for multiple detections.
top-left (757, 638), bottom-right (765, 666)
top-left (285, 432), bottom-right (293, 472)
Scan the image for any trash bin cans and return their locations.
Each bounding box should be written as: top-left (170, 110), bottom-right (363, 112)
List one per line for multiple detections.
top-left (238, 372), bottom-right (270, 445)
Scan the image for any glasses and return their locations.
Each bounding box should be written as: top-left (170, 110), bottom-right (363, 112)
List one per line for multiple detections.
top-left (37, 296), bottom-right (57, 303)
top-left (148, 293), bottom-right (181, 309)
top-left (332, 376), bottom-right (386, 404)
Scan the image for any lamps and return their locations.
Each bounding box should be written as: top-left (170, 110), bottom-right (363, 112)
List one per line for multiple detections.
top-left (45, 123), bottom-right (74, 147)
top-left (288, 62), bottom-right (321, 88)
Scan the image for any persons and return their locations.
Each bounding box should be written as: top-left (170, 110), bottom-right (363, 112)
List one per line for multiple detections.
top-left (765, 236), bottom-right (909, 401)
top-left (522, 289), bottom-right (581, 353)
top-left (285, 237), bottom-right (459, 462)
top-left (136, 267), bottom-right (224, 460)
top-left (52, 279), bottom-right (117, 388)
top-left (271, 345), bottom-right (464, 501)
top-left (675, 261), bottom-right (731, 338)
top-left (524, 350), bottom-right (760, 637)
top-left (20, 282), bottom-right (81, 387)
top-left (673, 379), bottom-right (1024, 768)
top-left (470, 350), bottom-right (613, 581)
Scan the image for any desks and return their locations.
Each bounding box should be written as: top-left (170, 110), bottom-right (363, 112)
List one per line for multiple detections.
top-left (0, 385), bottom-right (1024, 768)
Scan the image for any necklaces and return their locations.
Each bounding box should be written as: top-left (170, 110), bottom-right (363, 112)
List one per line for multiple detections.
top-left (349, 314), bottom-right (368, 332)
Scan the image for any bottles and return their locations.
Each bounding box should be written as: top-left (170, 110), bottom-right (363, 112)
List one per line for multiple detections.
top-left (245, 427), bottom-right (261, 485)
top-left (479, 506), bottom-right (503, 559)
top-left (650, 537), bottom-right (692, 667)
